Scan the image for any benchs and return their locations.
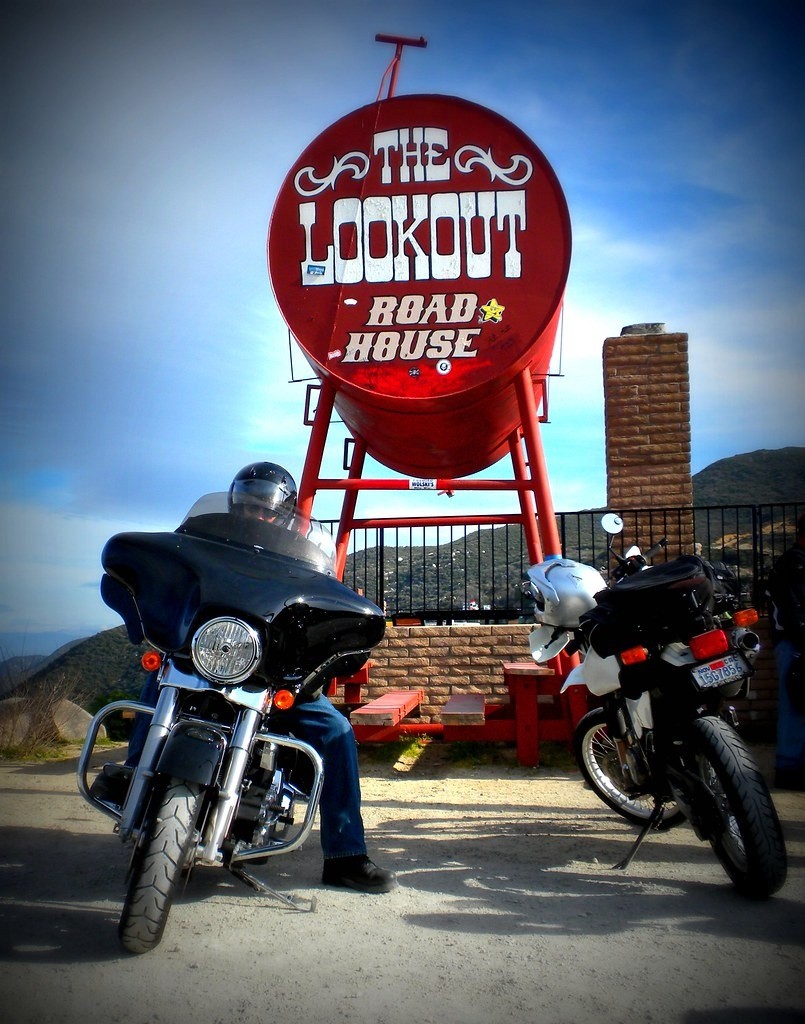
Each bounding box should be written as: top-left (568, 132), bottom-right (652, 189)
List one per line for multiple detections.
top-left (502, 662), bottom-right (555, 767)
top-left (327, 662), bottom-right (373, 704)
top-left (350, 690), bottom-right (424, 742)
top-left (440, 695), bottom-right (485, 742)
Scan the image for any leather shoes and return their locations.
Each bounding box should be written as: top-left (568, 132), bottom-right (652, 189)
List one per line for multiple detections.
top-left (89, 762), bottom-right (135, 805)
top-left (321, 853), bottom-right (398, 894)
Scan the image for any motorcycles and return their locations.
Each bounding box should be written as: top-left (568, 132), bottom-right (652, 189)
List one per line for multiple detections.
top-left (74, 490), bottom-right (386, 957)
top-left (521, 513), bottom-right (786, 901)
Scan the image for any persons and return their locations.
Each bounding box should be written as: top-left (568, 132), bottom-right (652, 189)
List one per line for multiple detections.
top-left (103, 462), bottom-right (396, 893)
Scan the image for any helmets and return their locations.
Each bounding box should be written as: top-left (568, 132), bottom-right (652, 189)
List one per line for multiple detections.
top-left (520, 559), bottom-right (612, 629)
top-left (227, 461), bottom-right (299, 529)
